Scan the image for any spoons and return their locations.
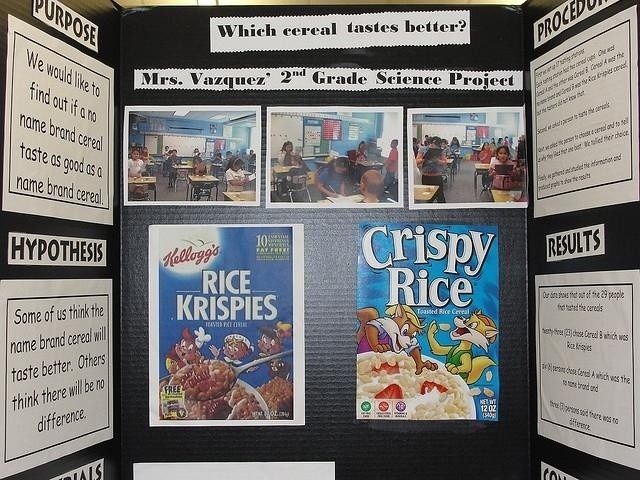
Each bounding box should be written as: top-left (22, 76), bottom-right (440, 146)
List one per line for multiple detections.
top-left (165, 346), bottom-right (292, 403)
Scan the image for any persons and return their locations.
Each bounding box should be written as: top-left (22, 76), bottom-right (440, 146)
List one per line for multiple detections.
top-left (278, 141), bottom-right (298, 199)
top-left (317, 157), bottom-right (352, 198)
top-left (355, 140), bottom-right (369, 165)
top-left (128, 143), bottom-right (257, 192)
top-left (384, 140), bottom-right (398, 191)
top-left (360, 170), bottom-right (383, 204)
top-left (285, 154), bottom-right (311, 201)
top-left (413, 135), bottom-right (525, 203)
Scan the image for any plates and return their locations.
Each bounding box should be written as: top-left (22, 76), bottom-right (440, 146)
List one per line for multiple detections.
top-left (160, 372), bottom-right (273, 420)
top-left (357, 349), bottom-right (478, 420)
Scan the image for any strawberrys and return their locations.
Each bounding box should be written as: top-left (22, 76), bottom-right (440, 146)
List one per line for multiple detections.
top-left (420, 381), bottom-right (448, 395)
top-left (375, 384), bottom-right (403, 399)
top-left (371, 361), bottom-right (400, 375)
